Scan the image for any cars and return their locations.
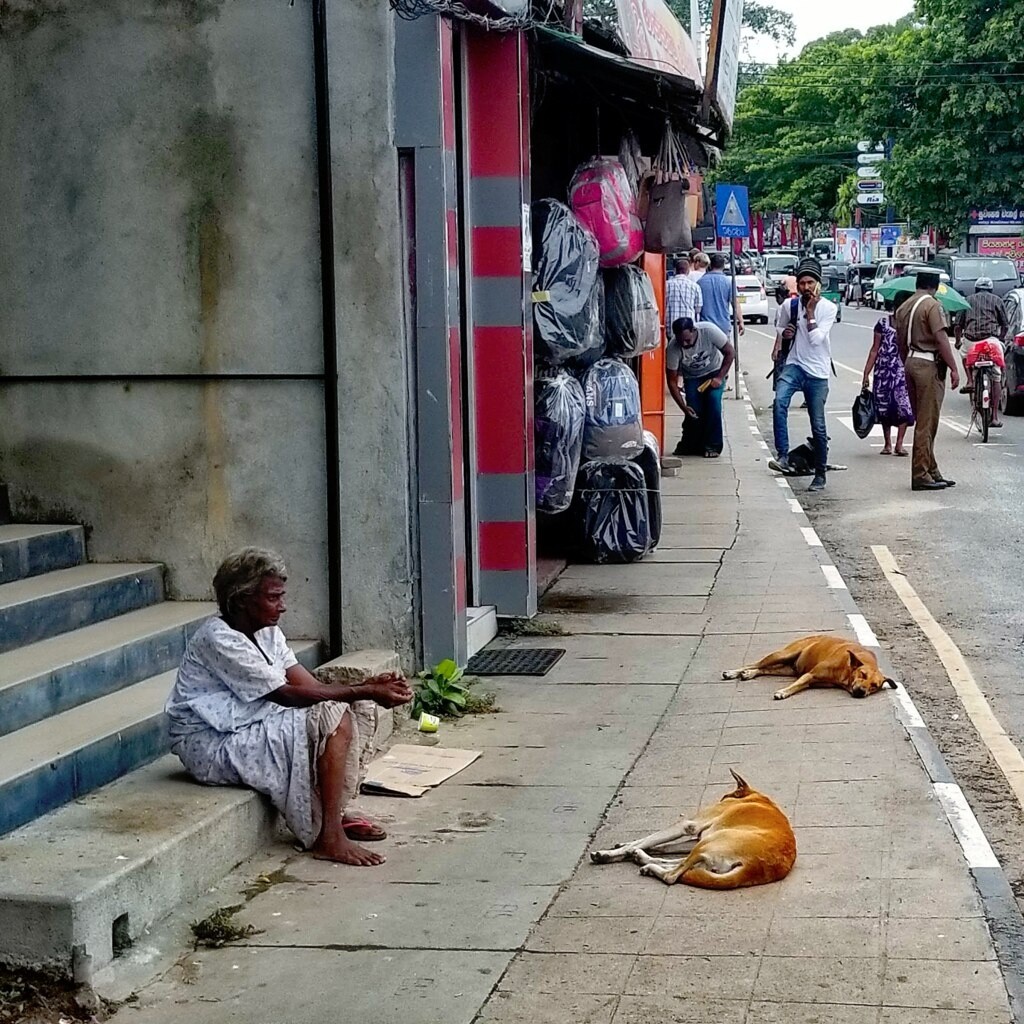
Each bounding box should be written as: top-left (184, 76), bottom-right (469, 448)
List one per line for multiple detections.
top-left (998, 288), bottom-right (1024, 416)
top-left (727, 274), bottom-right (769, 325)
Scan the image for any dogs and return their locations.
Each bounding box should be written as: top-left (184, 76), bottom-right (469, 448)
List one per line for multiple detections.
top-left (589, 768), bottom-right (797, 891)
top-left (783, 436), bottom-right (847, 477)
top-left (722, 636), bottom-right (899, 700)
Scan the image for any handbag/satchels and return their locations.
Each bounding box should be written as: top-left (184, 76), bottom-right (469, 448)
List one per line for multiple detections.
top-left (773, 299), bottom-right (798, 390)
top-left (641, 122), bottom-right (713, 254)
top-left (852, 387), bottom-right (875, 439)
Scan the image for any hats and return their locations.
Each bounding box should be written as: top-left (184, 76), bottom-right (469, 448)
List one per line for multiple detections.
top-left (975, 278), bottom-right (993, 289)
top-left (913, 267), bottom-right (945, 276)
top-left (795, 259), bottom-right (822, 283)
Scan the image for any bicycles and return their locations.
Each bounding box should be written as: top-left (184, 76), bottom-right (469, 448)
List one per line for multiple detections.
top-left (960, 334), bottom-right (1000, 443)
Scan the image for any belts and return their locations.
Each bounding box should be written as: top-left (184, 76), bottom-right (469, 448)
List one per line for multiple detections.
top-left (906, 350), bottom-right (934, 361)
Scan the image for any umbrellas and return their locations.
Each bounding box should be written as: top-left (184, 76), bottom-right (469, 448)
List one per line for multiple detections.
top-left (872, 276), bottom-right (972, 311)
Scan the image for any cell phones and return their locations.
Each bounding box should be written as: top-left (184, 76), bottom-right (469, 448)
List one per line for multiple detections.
top-left (813, 282), bottom-right (822, 297)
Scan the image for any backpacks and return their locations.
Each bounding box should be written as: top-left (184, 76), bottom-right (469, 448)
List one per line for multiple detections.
top-left (530, 147), bottom-right (663, 563)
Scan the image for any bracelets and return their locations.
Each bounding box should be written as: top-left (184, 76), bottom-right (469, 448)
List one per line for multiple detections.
top-left (808, 319), bottom-right (816, 325)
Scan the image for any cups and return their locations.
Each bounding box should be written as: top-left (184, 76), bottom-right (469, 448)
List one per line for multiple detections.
top-left (417, 711), bottom-right (439, 731)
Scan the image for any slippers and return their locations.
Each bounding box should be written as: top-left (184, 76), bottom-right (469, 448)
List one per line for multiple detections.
top-left (990, 422), bottom-right (1003, 427)
top-left (959, 386), bottom-right (973, 395)
top-left (702, 449), bottom-right (718, 458)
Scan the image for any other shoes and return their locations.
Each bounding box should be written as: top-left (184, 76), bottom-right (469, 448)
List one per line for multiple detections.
top-left (917, 480), bottom-right (947, 491)
top-left (936, 479), bottom-right (956, 487)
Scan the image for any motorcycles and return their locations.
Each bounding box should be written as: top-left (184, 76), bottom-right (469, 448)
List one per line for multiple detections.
top-left (821, 265), bottom-right (844, 322)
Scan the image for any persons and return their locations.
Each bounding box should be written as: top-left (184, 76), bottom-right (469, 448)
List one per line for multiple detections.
top-left (688, 246), bottom-right (711, 282)
top-left (695, 255), bottom-right (745, 390)
top-left (768, 263), bottom-right (837, 492)
top-left (666, 260), bottom-right (703, 346)
top-left (896, 268), bottom-right (960, 490)
top-left (847, 270), bottom-right (862, 309)
top-left (665, 317), bottom-right (736, 458)
top-left (954, 276), bottom-right (1008, 428)
top-left (167, 544), bottom-right (415, 867)
top-left (862, 291), bottom-right (914, 455)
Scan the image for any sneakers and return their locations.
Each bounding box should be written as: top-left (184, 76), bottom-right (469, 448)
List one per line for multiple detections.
top-left (768, 455), bottom-right (790, 473)
top-left (808, 475), bottom-right (826, 492)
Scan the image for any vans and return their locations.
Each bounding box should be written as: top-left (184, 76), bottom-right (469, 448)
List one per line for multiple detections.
top-left (664, 222), bottom-right (1024, 337)
top-left (754, 253), bottom-right (800, 295)
